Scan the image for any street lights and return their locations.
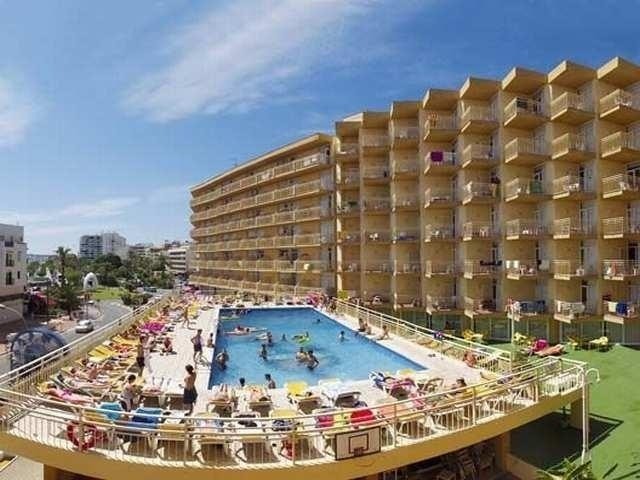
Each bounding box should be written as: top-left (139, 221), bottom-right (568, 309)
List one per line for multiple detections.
top-left (574, 365), bottom-right (601, 477)
top-left (0, 304), bottom-right (31, 332)
top-left (505, 301), bottom-right (522, 341)
top-left (86, 300), bottom-right (94, 319)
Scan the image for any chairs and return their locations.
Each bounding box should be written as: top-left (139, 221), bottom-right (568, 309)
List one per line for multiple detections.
top-left (204, 293), bottom-right (304, 307)
top-left (27, 294), bottom-right (205, 412)
top-left (81, 372), bottom-right (518, 456)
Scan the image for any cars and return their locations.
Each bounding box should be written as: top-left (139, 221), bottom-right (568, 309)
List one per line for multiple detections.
top-left (75, 319), bottom-right (94, 333)
top-left (32, 286), bottom-right (40, 291)
top-left (134, 287), bottom-right (157, 297)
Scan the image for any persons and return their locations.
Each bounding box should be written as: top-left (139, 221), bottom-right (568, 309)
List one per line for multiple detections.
top-left (216, 348), bottom-right (230, 372)
top-left (243, 327), bottom-right (255, 334)
top-left (39, 288), bottom-right (214, 416)
top-left (293, 345), bottom-right (307, 361)
top-left (355, 318), bottom-right (365, 333)
top-left (333, 330), bottom-right (351, 345)
top-left (238, 377), bottom-right (249, 385)
top-left (214, 380), bottom-right (231, 403)
top-left (444, 375), bottom-right (474, 398)
top-left (248, 383), bottom-right (270, 406)
top-left (263, 330), bottom-right (275, 348)
top-left (286, 389), bottom-right (316, 405)
top-left (306, 351), bottom-right (320, 371)
top-left (278, 332), bottom-right (287, 342)
top-left (233, 322), bottom-right (242, 334)
top-left (292, 330), bottom-right (311, 346)
top-left (372, 325), bottom-right (395, 342)
top-left (310, 318), bottom-right (326, 325)
top-left (362, 323), bottom-right (373, 336)
top-left (256, 343), bottom-right (269, 362)
top-left (219, 291), bottom-right (344, 317)
top-left (262, 374), bottom-right (278, 389)
